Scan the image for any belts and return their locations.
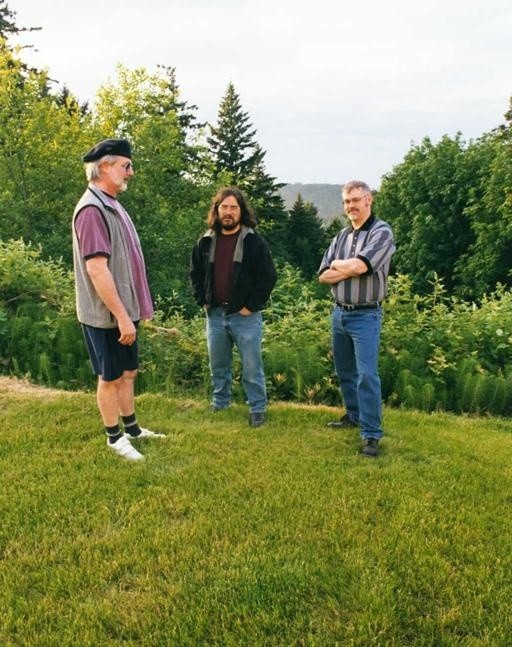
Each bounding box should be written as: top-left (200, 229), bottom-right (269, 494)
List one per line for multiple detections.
top-left (336, 302), bottom-right (377, 309)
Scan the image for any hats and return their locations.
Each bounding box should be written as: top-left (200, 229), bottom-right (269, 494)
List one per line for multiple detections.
top-left (82, 138), bottom-right (132, 162)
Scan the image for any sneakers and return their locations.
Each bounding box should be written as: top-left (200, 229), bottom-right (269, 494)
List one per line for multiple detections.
top-left (107, 436), bottom-right (142, 457)
top-left (362, 439), bottom-right (378, 455)
top-left (125, 426), bottom-right (165, 438)
top-left (326, 415), bottom-right (357, 427)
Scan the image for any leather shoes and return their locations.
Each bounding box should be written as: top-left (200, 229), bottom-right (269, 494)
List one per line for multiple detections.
top-left (250, 413), bottom-right (264, 426)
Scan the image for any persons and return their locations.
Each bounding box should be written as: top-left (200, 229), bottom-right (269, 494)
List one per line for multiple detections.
top-left (71, 139), bottom-right (166, 461)
top-left (191, 185), bottom-right (277, 428)
top-left (317, 180), bottom-right (396, 457)
